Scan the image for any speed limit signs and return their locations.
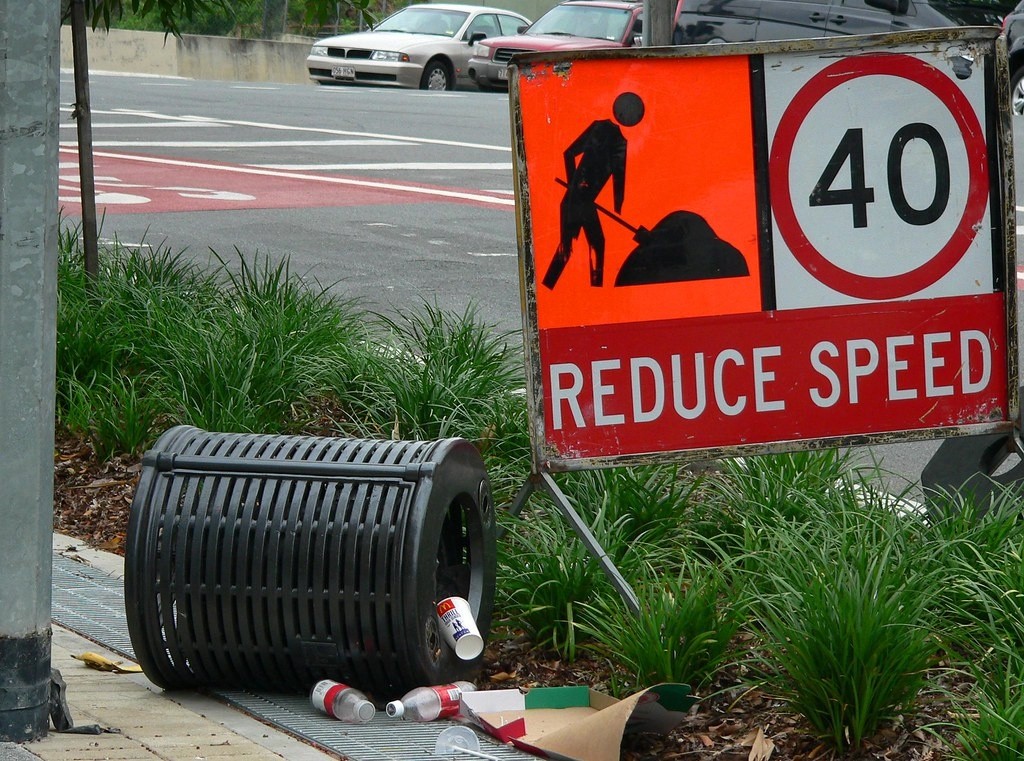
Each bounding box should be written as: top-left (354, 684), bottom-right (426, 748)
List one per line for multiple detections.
top-left (769, 50), bottom-right (988, 299)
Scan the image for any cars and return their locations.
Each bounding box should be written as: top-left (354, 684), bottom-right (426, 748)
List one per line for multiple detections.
top-left (466, 0), bottom-right (648, 94)
top-left (1002, 0), bottom-right (1024, 117)
top-left (307, 4), bottom-right (533, 91)
top-left (670, 0), bottom-right (972, 47)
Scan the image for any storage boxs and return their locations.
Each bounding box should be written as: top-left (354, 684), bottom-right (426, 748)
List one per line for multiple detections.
top-left (460, 682), bottom-right (702, 761)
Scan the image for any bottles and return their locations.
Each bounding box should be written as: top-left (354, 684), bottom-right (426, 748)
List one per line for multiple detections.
top-left (386, 680), bottom-right (478, 722)
top-left (308, 679), bottom-right (376, 726)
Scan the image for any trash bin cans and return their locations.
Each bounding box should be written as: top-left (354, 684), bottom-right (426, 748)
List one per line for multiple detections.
top-left (124, 424), bottom-right (498, 699)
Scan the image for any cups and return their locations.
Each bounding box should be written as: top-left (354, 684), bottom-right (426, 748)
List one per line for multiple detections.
top-left (435, 596), bottom-right (483, 661)
